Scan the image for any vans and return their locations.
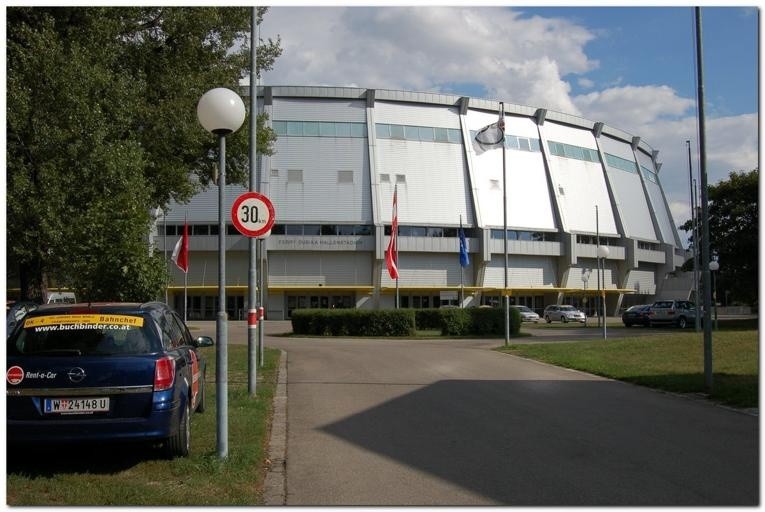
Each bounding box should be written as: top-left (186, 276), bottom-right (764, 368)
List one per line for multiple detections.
top-left (47, 292), bottom-right (77, 305)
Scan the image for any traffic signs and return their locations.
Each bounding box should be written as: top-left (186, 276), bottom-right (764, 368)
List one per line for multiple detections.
top-left (231, 192), bottom-right (275, 237)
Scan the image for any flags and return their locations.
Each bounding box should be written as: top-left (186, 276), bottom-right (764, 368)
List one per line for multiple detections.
top-left (386, 191), bottom-right (399, 280)
top-left (459, 223), bottom-right (469, 268)
top-left (170, 222), bottom-right (188, 273)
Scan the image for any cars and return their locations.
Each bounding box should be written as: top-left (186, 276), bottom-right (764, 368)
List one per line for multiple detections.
top-left (8, 302), bottom-right (214, 457)
top-left (510, 305), bottom-right (539, 324)
top-left (622, 300), bottom-right (704, 329)
top-left (543, 305), bottom-right (587, 323)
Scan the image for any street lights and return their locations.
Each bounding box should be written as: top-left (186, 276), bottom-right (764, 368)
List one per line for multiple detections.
top-left (709, 261), bottom-right (719, 331)
top-left (197, 88), bottom-right (245, 455)
top-left (597, 245), bottom-right (609, 339)
